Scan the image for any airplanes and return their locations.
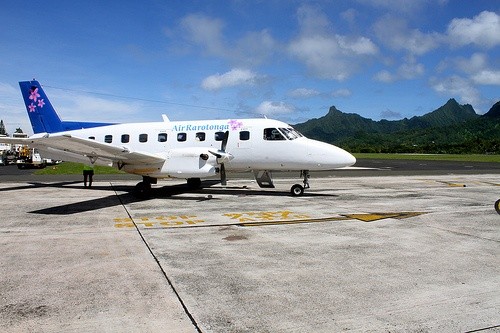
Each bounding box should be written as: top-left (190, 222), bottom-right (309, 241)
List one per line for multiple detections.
top-left (0, 78), bottom-right (357, 189)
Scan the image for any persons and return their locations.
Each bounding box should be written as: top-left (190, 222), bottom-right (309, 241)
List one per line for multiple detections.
top-left (83, 164), bottom-right (95, 189)
